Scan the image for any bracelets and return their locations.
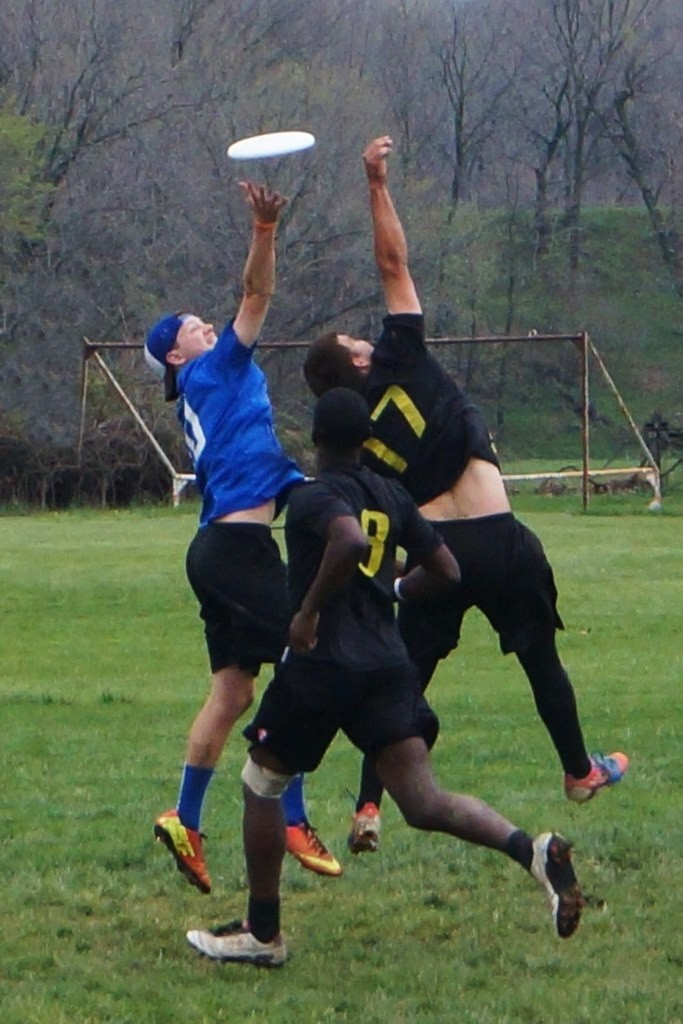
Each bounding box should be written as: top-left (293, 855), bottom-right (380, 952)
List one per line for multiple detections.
top-left (253, 218), bottom-right (274, 230)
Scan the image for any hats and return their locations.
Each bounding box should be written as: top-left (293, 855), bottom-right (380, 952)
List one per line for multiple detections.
top-left (144, 310), bottom-right (193, 402)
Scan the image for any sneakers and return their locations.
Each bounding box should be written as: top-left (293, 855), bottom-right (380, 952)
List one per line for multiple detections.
top-left (285, 817), bottom-right (342, 876)
top-left (564, 749), bottom-right (628, 802)
top-left (188, 920), bottom-right (286, 968)
top-left (531, 832), bottom-right (581, 941)
top-left (154, 810), bottom-right (212, 894)
top-left (348, 802), bottom-right (380, 854)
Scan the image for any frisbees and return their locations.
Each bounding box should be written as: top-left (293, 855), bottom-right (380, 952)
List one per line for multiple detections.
top-left (223, 129), bottom-right (319, 162)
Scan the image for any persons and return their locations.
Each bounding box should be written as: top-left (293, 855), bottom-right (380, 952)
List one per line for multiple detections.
top-left (185, 388), bottom-right (583, 965)
top-left (303, 136), bottom-right (631, 854)
top-left (143, 178), bottom-right (342, 891)
